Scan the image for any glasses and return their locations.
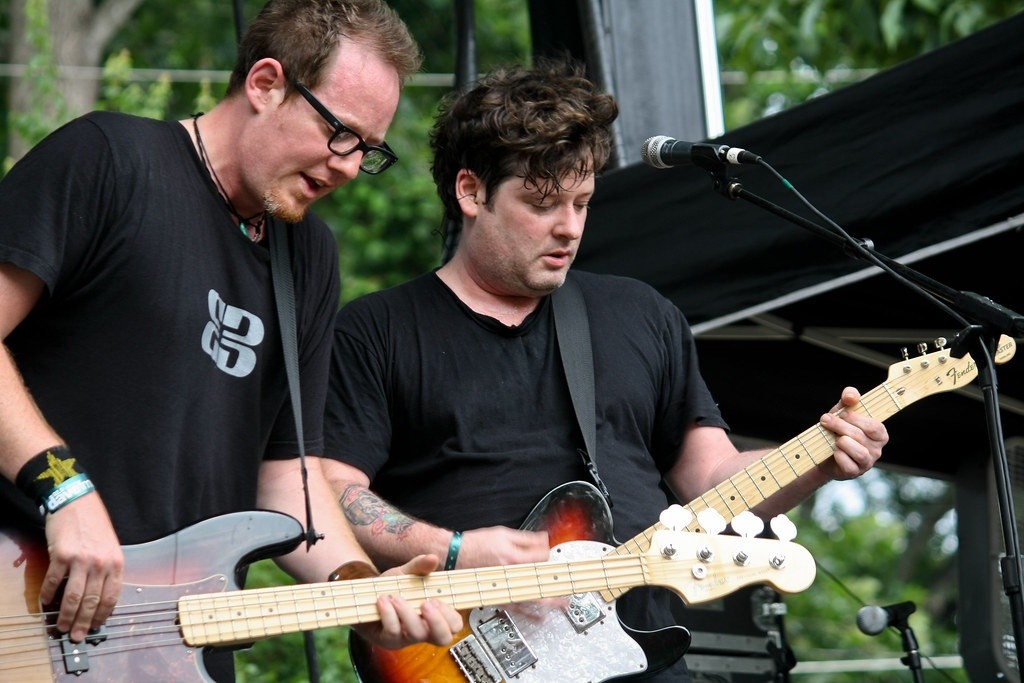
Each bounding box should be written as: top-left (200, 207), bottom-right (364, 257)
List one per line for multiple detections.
top-left (284, 67), bottom-right (399, 175)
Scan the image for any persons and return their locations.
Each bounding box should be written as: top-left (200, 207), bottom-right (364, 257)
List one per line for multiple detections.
top-left (317, 71), bottom-right (890, 683)
top-left (0, 0), bottom-right (466, 683)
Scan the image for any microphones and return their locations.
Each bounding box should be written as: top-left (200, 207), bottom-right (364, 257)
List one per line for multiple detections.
top-left (856, 601), bottom-right (916, 636)
top-left (640, 136), bottom-right (762, 169)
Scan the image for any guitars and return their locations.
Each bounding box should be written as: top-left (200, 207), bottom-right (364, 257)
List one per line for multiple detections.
top-left (0, 505), bottom-right (817, 683)
top-left (345, 331), bottom-right (1016, 683)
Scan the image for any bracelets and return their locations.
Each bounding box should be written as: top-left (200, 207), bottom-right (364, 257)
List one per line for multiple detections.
top-left (11, 444), bottom-right (86, 518)
top-left (445, 530), bottom-right (462, 571)
top-left (40, 472), bottom-right (95, 515)
top-left (329, 561), bottom-right (381, 582)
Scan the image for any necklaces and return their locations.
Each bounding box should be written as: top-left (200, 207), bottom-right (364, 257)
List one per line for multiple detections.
top-left (193, 111), bottom-right (267, 244)
